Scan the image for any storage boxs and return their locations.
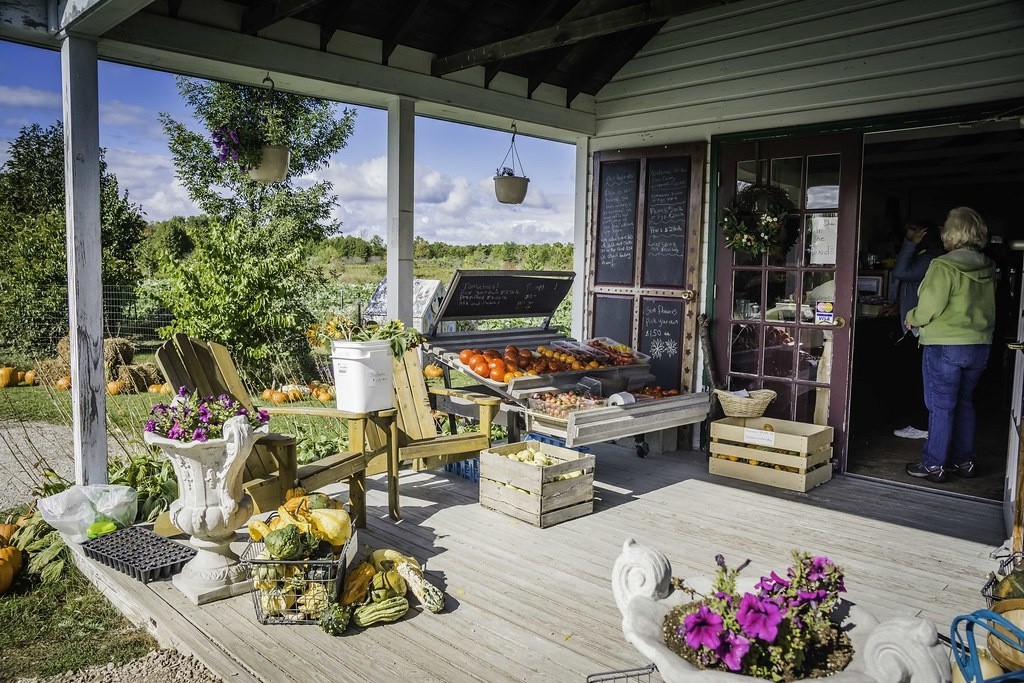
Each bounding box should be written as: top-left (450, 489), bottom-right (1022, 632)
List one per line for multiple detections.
top-left (479, 441), bottom-right (598, 526)
top-left (524, 383), bottom-right (709, 447)
top-left (710, 417), bottom-right (833, 491)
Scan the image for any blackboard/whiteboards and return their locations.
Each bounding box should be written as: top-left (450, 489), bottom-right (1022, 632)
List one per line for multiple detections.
top-left (437, 268), bottom-right (577, 320)
top-left (593, 158), bottom-right (640, 287)
top-left (591, 291), bottom-right (635, 348)
top-left (637, 294), bottom-right (685, 394)
top-left (640, 154), bottom-right (692, 292)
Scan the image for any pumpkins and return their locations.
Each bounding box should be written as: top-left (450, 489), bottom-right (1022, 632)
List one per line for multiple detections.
top-left (261, 379), bottom-right (336, 402)
top-left (0, 499), bottom-right (39, 596)
top-left (148, 382), bottom-right (172, 394)
top-left (0, 364), bottom-right (72, 390)
top-left (108, 379), bottom-right (125, 395)
top-left (717, 424), bottom-right (829, 474)
top-left (425, 361), bottom-right (444, 378)
top-left (248, 482), bottom-right (445, 635)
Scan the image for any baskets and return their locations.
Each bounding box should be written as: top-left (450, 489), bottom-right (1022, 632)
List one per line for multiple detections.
top-left (714, 388), bottom-right (777, 418)
top-left (237, 511), bottom-right (358, 623)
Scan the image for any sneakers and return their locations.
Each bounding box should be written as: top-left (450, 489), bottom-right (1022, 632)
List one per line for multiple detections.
top-left (894, 426), bottom-right (929, 439)
top-left (944, 456), bottom-right (976, 478)
top-left (907, 461), bottom-right (947, 483)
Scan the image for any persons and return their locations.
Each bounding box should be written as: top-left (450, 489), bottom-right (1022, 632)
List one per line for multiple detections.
top-left (904, 207), bottom-right (996, 483)
top-left (879, 216), bottom-right (934, 440)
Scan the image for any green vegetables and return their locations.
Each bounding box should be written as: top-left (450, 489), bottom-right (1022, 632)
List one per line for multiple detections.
top-left (442, 423), bottom-right (509, 441)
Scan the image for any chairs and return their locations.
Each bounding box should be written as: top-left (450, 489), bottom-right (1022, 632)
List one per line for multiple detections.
top-left (332, 318), bottom-right (497, 519)
top-left (155, 333), bottom-right (378, 527)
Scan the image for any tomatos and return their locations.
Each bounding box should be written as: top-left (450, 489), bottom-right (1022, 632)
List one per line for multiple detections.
top-left (460, 339), bottom-right (679, 419)
top-left (732, 323), bottom-right (817, 378)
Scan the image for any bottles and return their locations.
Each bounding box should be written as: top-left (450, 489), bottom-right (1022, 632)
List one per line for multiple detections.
top-left (775, 303), bottom-right (811, 321)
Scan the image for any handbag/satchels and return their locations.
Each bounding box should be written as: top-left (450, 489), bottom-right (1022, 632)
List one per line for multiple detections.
top-left (889, 324), bottom-right (906, 351)
top-left (38, 484), bottom-right (138, 544)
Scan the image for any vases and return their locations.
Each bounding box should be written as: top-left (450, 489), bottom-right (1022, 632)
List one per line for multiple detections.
top-left (495, 177), bottom-right (529, 204)
top-left (142, 414), bottom-right (263, 602)
top-left (250, 145), bottom-right (292, 184)
top-left (609, 535), bottom-right (954, 683)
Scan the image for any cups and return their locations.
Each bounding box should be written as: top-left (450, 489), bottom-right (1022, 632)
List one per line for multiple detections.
top-left (736, 298), bottom-right (750, 319)
top-left (751, 302), bottom-right (759, 312)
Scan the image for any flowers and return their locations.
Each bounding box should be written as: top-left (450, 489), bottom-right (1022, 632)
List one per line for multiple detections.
top-left (666, 552), bottom-right (856, 676)
top-left (144, 388), bottom-right (264, 448)
top-left (718, 178), bottom-right (799, 265)
top-left (497, 166), bottom-right (527, 176)
top-left (208, 112), bottom-right (263, 173)
